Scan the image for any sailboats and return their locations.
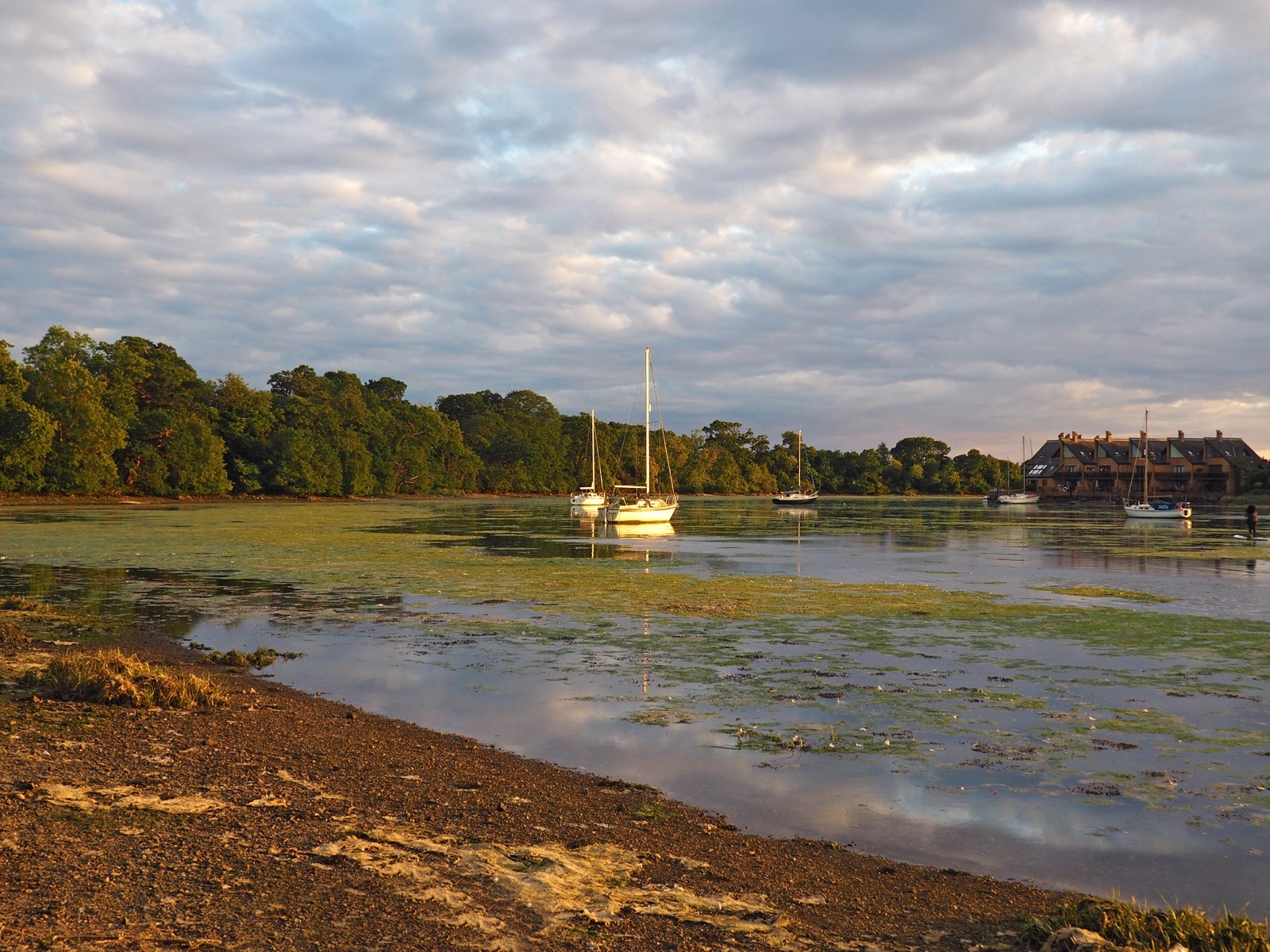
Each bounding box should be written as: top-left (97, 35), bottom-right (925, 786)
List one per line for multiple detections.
top-left (998, 436), bottom-right (1040, 504)
top-left (1122, 406), bottom-right (1192, 519)
top-left (570, 409), bottom-right (609, 506)
top-left (599, 346), bottom-right (680, 523)
top-left (772, 429), bottom-right (818, 504)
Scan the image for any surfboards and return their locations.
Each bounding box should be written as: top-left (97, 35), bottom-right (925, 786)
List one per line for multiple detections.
top-left (1232, 534), bottom-right (1270, 541)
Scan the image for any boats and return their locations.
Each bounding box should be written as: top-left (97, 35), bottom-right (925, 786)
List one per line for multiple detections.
top-left (1232, 534), bottom-right (1270, 541)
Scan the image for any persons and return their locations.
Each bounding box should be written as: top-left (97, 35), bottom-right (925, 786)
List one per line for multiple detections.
top-left (1244, 504), bottom-right (1258, 537)
